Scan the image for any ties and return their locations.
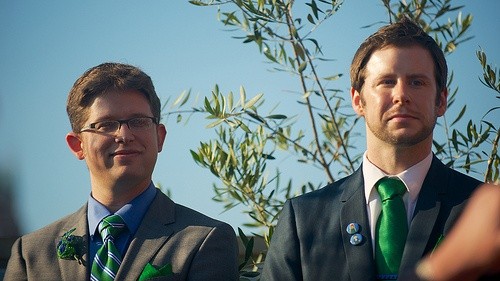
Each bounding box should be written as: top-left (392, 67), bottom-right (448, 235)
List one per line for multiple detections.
top-left (88, 213), bottom-right (127, 281)
top-left (374, 177), bottom-right (410, 275)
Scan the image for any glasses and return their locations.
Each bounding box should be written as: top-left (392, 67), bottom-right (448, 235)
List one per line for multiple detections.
top-left (78, 116), bottom-right (159, 136)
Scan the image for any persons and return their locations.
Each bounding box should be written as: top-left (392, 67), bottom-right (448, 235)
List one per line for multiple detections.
top-left (3, 62), bottom-right (241, 281)
top-left (259, 17), bottom-right (500, 281)
